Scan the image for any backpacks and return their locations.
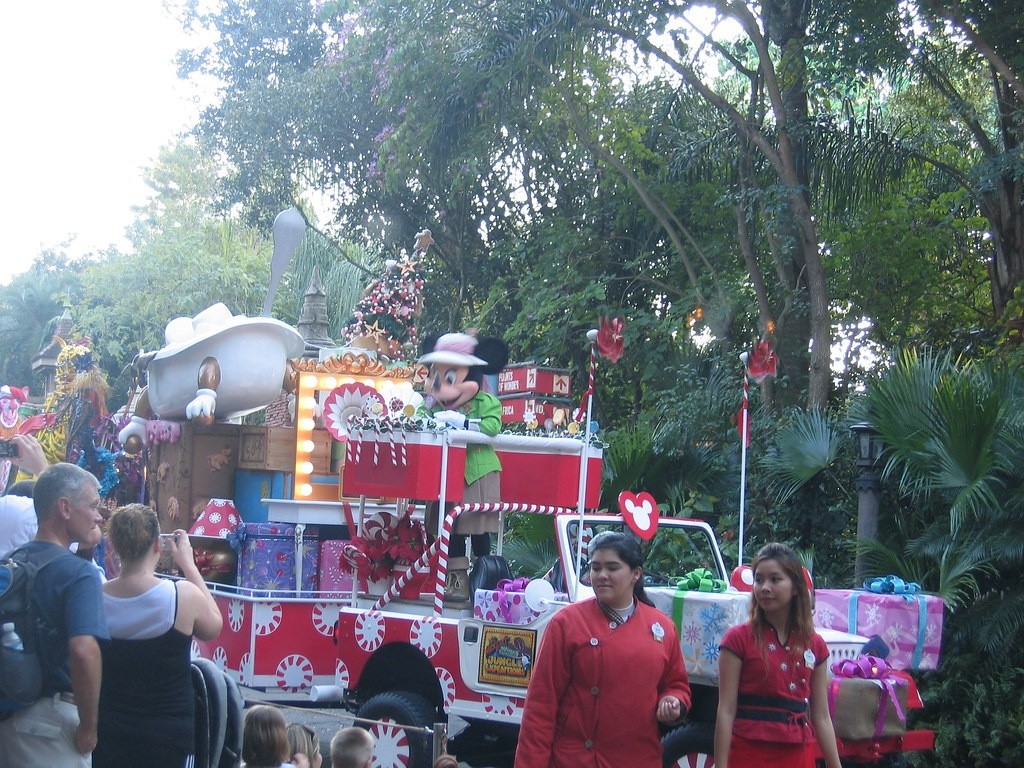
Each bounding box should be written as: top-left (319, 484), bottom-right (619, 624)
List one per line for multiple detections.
top-left (0, 546), bottom-right (75, 720)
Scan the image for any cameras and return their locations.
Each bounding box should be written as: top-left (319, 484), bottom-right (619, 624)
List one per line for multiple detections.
top-left (0, 440), bottom-right (19, 457)
top-left (160, 533), bottom-right (179, 552)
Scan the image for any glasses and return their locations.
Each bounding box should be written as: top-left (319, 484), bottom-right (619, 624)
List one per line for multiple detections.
top-left (290, 721), bottom-right (315, 743)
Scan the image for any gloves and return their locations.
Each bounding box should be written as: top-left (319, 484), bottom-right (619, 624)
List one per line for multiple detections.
top-left (434, 410), bottom-right (466, 430)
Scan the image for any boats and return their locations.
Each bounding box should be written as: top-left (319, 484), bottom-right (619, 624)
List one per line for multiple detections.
top-left (145, 302), bottom-right (307, 424)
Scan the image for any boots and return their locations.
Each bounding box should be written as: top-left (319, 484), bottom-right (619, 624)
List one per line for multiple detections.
top-left (445, 557), bottom-right (470, 600)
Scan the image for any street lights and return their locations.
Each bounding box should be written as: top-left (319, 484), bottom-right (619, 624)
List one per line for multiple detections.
top-left (849, 420), bottom-right (891, 587)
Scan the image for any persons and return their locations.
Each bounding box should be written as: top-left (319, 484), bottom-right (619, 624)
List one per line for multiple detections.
top-left (242, 705), bottom-right (291, 768)
top-left (515, 531), bottom-right (690, 768)
top-left (0, 463), bottom-right (102, 768)
top-left (418, 333), bottom-right (510, 602)
top-left (331, 727), bottom-right (376, 768)
top-left (287, 723), bottom-right (322, 768)
top-left (0, 434), bottom-right (106, 586)
top-left (715, 543), bottom-right (842, 768)
top-left (102, 503), bottom-right (223, 768)
